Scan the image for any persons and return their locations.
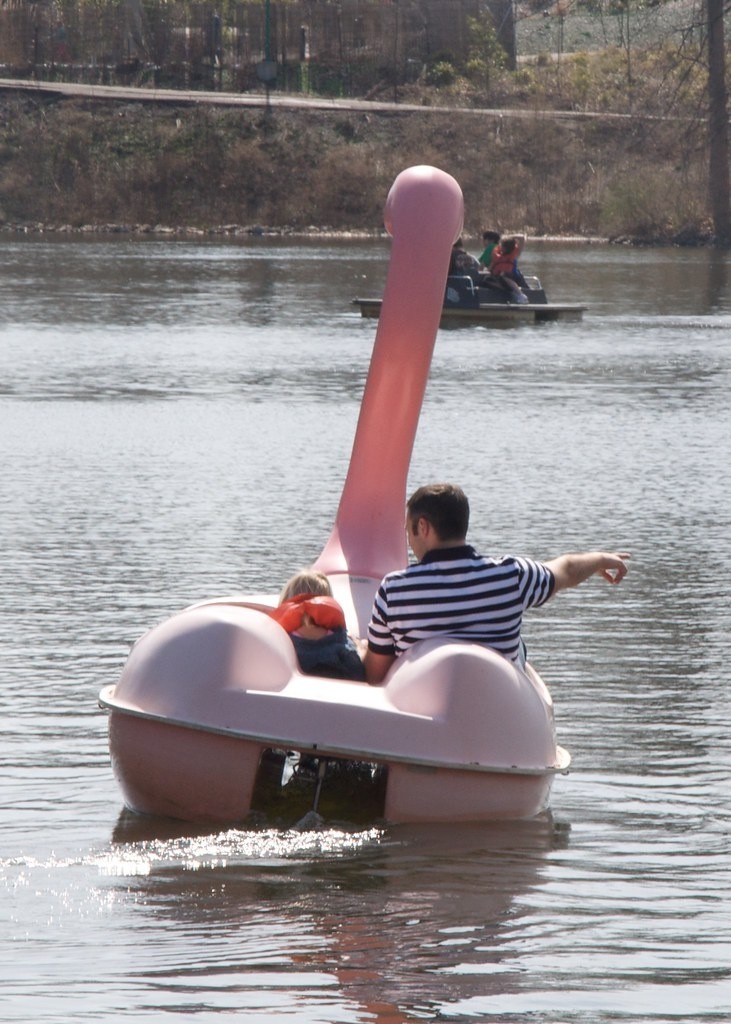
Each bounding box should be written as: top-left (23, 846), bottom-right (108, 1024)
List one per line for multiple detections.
top-left (490, 234), bottom-right (530, 305)
top-left (277, 571), bottom-right (366, 770)
top-left (476, 231), bottom-right (500, 269)
top-left (448, 237), bottom-right (480, 295)
top-left (362, 483), bottom-right (631, 687)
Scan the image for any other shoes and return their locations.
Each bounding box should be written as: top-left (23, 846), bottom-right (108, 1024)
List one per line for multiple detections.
top-left (513, 289), bottom-right (529, 303)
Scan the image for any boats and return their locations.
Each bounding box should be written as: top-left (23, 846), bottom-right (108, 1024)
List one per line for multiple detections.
top-left (352, 233), bottom-right (585, 331)
top-left (88, 163), bottom-right (576, 835)
